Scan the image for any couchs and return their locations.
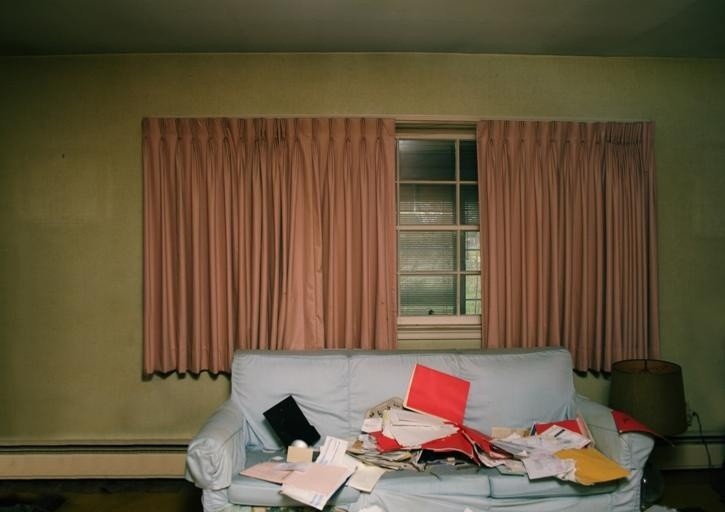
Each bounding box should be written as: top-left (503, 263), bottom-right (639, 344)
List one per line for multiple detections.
top-left (185, 349), bottom-right (654, 512)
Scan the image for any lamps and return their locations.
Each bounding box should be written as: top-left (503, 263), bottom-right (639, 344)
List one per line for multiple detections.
top-left (605, 358), bottom-right (688, 511)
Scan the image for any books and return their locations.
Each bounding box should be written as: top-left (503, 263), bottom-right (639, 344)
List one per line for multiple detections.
top-left (403, 364), bottom-right (469, 424)
top-left (554, 445), bottom-right (630, 488)
